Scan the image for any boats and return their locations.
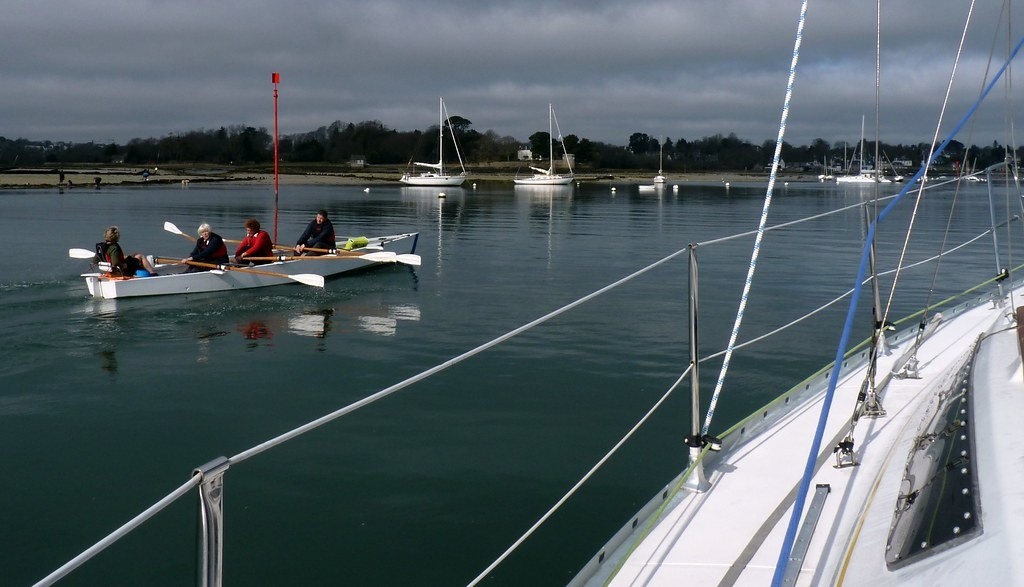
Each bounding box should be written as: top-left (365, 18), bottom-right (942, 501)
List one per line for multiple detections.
top-left (81, 231), bottom-right (419, 298)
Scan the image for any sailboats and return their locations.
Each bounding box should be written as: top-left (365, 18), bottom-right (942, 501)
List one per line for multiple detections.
top-left (513, 102), bottom-right (574, 185)
top-left (398, 97), bottom-right (468, 186)
top-left (653, 135), bottom-right (667, 182)
top-left (817, 115), bottom-right (988, 182)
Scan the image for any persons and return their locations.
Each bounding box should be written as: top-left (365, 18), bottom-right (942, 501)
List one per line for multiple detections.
top-left (92, 226), bottom-right (161, 276)
top-left (178, 224), bottom-right (229, 273)
top-left (234, 218), bottom-right (274, 267)
top-left (293, 210), bottom-right (336, 256)
top-left (94, 177), bottom-right (102, 189)
top-left (60, 169), bottom-right (65, 182)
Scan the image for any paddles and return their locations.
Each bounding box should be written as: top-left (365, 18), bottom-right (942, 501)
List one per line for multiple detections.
top-left (243, 251), bottom-right (398, 264)
top-left (304, 246), bottom-right (423, 267)
top-left (186, 260), bottom-right (325, 288)
top-left (380, 301), bottom-right (421, 322)
top-left (355, 314), bottom-right (396, 336)
top-left (222, 238), bottom-right (294, 250)
top-left (286, 314), bottom-right (325, 333)
top-left (66, 248), bottom-right (185, 264)
top-left (163, 221), bottom-right (197, 243)
